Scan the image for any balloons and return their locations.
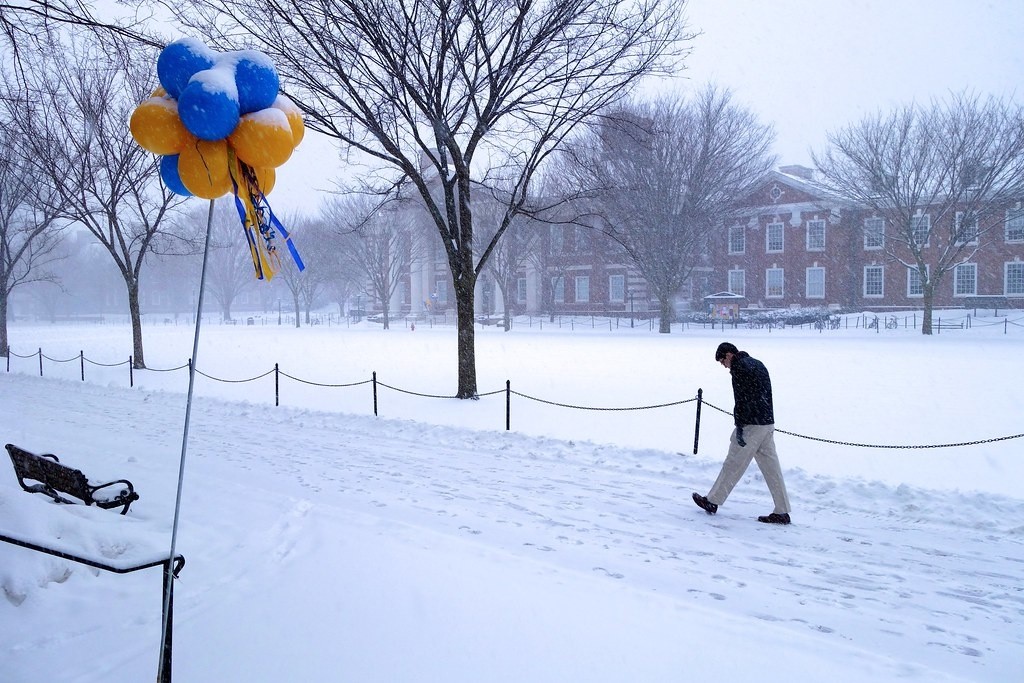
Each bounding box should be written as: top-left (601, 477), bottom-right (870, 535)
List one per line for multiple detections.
top-left (129, 38), bottom-right (305, 202)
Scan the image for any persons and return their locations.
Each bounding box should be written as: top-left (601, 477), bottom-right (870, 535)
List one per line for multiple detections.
top-left (692, 342), bottom-right (792, 524)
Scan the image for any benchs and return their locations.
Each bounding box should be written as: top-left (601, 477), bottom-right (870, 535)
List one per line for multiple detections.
top-left (5, 444), bottom-right (139, 515)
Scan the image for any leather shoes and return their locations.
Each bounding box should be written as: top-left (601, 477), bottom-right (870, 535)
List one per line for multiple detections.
top-left (691, 492), bottom-right (719, 513)
top-left (758, 512), bottom-right (790, 526)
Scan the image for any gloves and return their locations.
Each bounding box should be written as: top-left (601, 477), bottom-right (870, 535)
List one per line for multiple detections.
top-left (736, 428), bottom-right (747, 448)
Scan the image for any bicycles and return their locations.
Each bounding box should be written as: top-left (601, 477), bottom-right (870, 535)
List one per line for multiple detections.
top-left (869, 315), bottom-right (880, 328)
top-left (815, 316), bottom-right (841, 331)
top-left (887, 315), bottom-right (899, 328)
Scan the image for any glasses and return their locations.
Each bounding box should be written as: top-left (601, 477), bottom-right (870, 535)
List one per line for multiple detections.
top-left (721, 357), bottom-right (729, 364)
